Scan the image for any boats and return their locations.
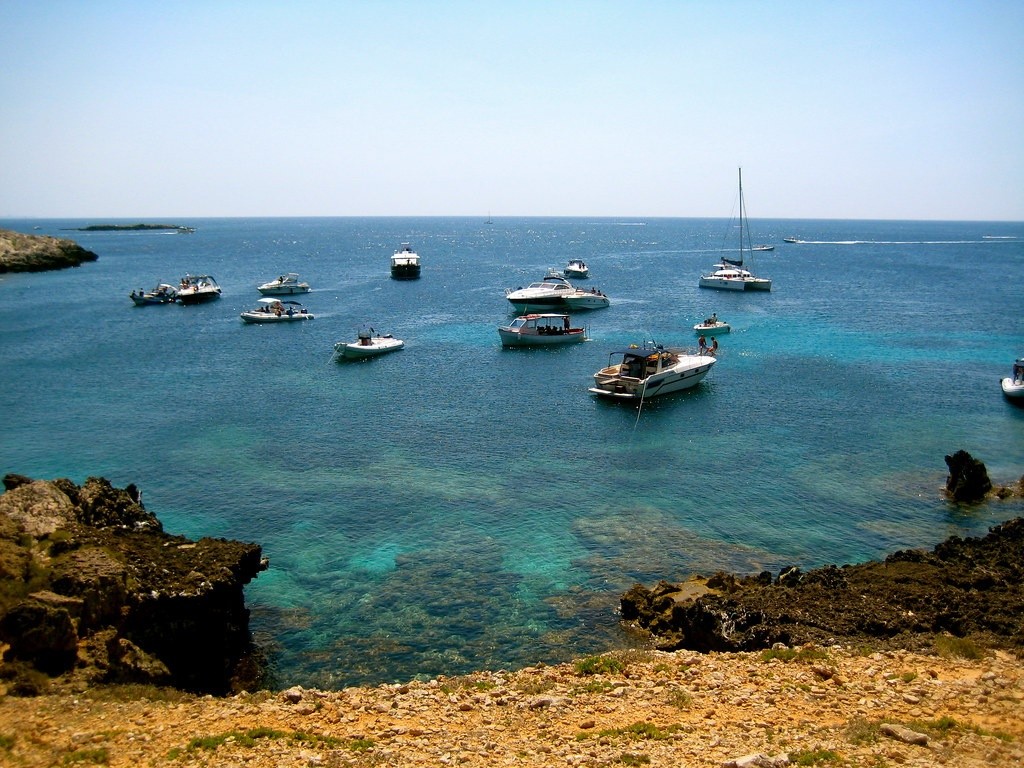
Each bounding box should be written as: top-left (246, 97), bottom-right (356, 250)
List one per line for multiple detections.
top-left (999, 357), bottom-right (1024, 398)
top-left (335, 328), bottom-right (405, 359)
top-left (587, 332), bottom-right (718, 400)
top-left (390, 242), bottom-right (421, 280)
top-left (751, 242), bottom-right (774, 252)
top-left (783, 237), bottom-right (798, 244)
top-left (698, 168), bottom-right (773, 294)
top-left (130, 284), bottom-right (178, 305)
top-left (564, 260), bottom-right (590, 279)
top-left (178, 273), bottom-right (222, 304)
top-left (503, 267), bottom-right (611, 314)
top-left (693, 319), bottom-right (732, 336)
top-left (256, 272), bottom-right (311, 296)
top-left (242, 298), bottom-right (316, 325)
top-left (496, 313), bottom-right (594, 345)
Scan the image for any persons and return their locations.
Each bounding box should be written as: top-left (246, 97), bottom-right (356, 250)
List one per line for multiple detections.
top-left (1012, 358), bottom-right (1024, 387)
top-left (711, 313), bottom-right (718, 322)
top-left (536, 325), bottom-right (562, 336)
top-left (745, 266), bottom-right (749, 271)
top-left (563, 316), bottom-right (572, 335)
top-left (278, 276), bottom-right (283, 284)
top-left (698, 334), bottom-right (706, 355)
top-left (590, 287), bottom-right (596, 296)
top-left (139, 288), bottom-right (145, 298)
top-left (162, 287), bottom-right (168, 295)
top-left (727, 274), bottom-right (731, 279)
top-left (703, 319), bottom-right (709, 327)
top-left (596, 289), bottom-right (603, 296)
top-left (180, 271), bottom-right (192, 287)
top-left (285, 307), bottom-right (295, 320)
top-left (131, 289), bottom-right (138, 297)
top-left (258, 304), bottom-right (284, 318)
top-left (704, 337), bottom-right (718, 355)
